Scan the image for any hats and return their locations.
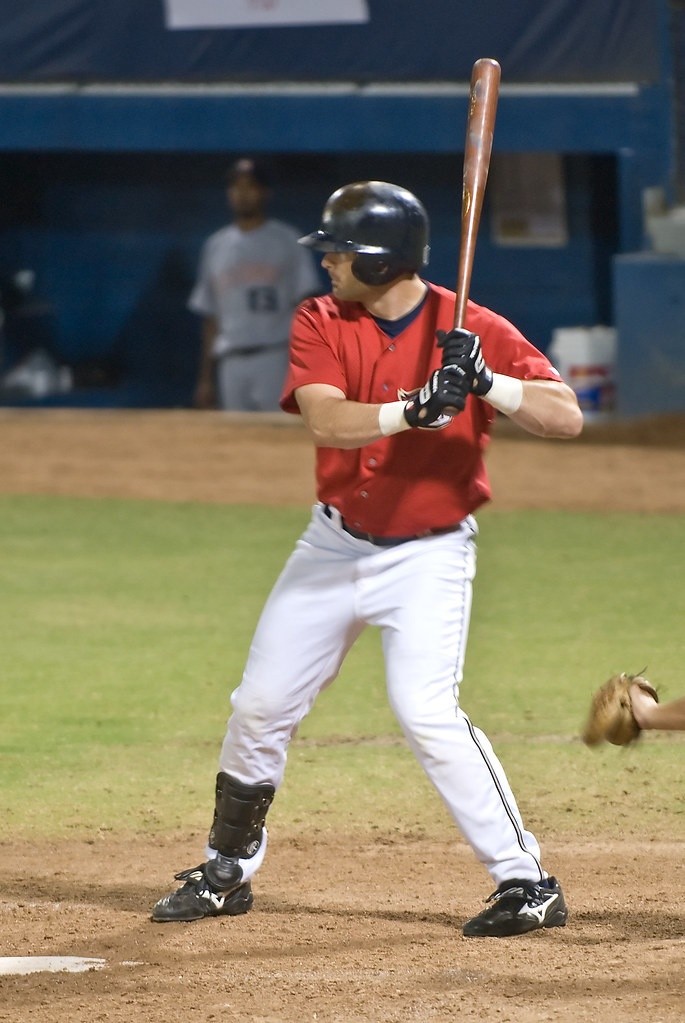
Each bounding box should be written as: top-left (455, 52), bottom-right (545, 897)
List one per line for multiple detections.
top-left (226, 157), bottom-right (267, 180)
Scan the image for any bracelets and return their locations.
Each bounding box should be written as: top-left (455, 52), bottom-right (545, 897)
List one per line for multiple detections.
top-left (478, 372), bottom-right (523, 414)
top-left (379, 401), bottom-right (413, 437)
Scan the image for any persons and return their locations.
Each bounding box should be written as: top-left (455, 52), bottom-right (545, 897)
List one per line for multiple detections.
top-left (628, 683), bottom-right (685, 731)
top-left (152, 180), bottom-right (584, 937)
top-left (188, 159), bottom-right (318, 412)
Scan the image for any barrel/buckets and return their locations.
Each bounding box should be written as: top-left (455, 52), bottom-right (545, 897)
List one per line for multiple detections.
top-left (552, 328), bottom-right (619, 424)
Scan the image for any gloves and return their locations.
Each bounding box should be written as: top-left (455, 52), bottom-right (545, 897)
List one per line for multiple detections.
top-left (436, 327), bottom-right (494, 396)
top-left (403, 365), bottom-right (470, 432)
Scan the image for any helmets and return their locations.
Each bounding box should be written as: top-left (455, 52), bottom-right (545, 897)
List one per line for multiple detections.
top-left (297, 180), bottom-right (430, 284)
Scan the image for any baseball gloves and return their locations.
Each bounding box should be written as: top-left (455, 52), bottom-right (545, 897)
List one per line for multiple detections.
top-left (584, 673), bottom-right (659, 749)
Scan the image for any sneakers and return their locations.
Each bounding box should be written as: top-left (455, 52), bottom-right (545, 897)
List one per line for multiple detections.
top-left (151, 860), bottom-right (254, 922)
top-left (463, 876), bottom-right (567, 936)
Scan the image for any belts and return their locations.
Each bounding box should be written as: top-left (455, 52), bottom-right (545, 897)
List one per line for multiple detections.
top-left (322, 504), bottom-right (461, 547)
top-left (214, 345), bottom-right (258, 362)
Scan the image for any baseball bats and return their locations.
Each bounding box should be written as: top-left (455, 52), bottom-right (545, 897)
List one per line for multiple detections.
top-left (440, 57), bottom-right (502, 418)
